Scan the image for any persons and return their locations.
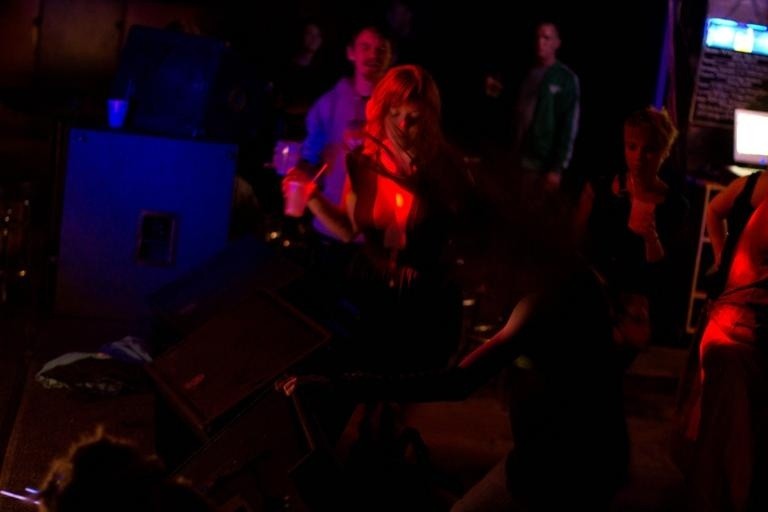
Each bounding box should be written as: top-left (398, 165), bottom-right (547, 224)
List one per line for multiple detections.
top-left (39, 3), bottom-right (697, 511)
top-left (699, 169), bottom-right (768, 510)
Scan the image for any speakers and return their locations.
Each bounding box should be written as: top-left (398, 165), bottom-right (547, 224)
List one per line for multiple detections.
top-left (55, 121), bottom-right (240, 323)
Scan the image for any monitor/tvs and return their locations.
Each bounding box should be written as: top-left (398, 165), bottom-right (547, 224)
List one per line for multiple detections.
top-left (732, 108), bottom-right (768, 165)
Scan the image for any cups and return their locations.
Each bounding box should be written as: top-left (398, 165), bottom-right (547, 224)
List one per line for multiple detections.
top-left (284, 184), bottom-right (305, 217)
top-left (275, 141), bottom-right (300, 176)
top-left (107, 98), bottom-right (129, 129)
